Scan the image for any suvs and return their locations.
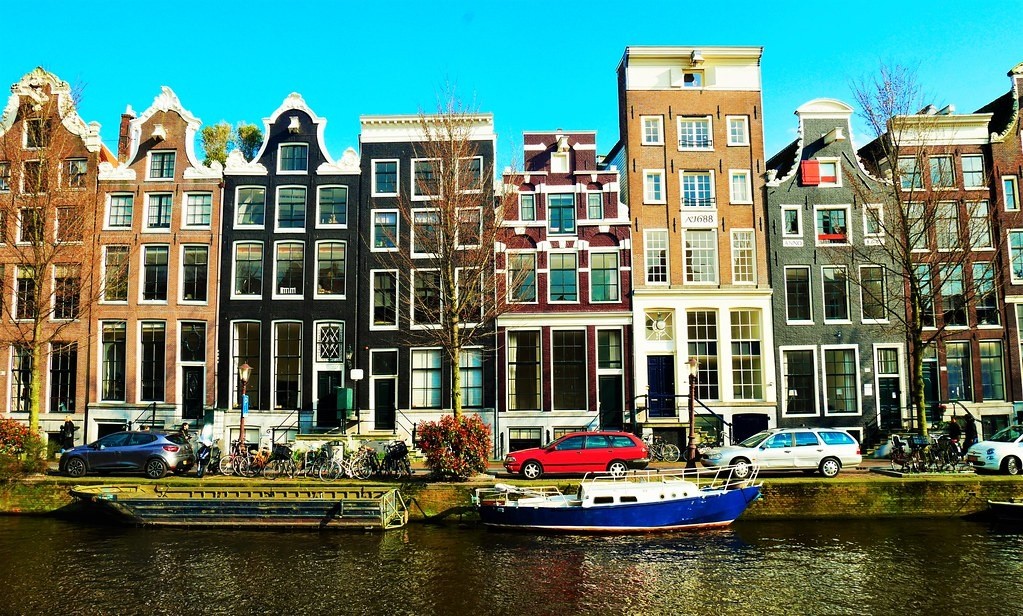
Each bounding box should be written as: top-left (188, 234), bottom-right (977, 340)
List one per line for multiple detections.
top-left (59, 430), bottom-right (196, 479)
top-left (503, 429), bottom-right (649, 480)
top-left (700, 427), bottom-right (862, 480)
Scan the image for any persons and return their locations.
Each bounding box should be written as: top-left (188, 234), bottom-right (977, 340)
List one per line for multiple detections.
top-left (963, 414), bottom-right (978, 453)
top-left (949, 417), bottom-right (961, 444)
top-left (179, 423), bottom-right (193, 441)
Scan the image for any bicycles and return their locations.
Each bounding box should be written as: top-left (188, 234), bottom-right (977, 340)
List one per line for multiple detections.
top-left (891, 433), bottom-right (964, 473)
top-left (644, 433), bottom-right (688, 463)
top-left (195, 438), bottom-right (416, 482)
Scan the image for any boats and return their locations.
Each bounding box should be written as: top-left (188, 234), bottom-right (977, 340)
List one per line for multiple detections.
top-left (465, 460), bottom-right (772, 535)
top-left (987, 498), bottom-right (1023, 518)
top-left (66, 482), bottom-right (409, 535)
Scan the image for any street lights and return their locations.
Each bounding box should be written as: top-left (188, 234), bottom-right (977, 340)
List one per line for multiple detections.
top-left (684, 356), bottom-right (701, 478)
top-left (236, 361), bottom-right (254, 449)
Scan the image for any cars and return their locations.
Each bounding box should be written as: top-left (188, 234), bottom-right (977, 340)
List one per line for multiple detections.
top-left (965, 424), bottom-right (1023, 476)
